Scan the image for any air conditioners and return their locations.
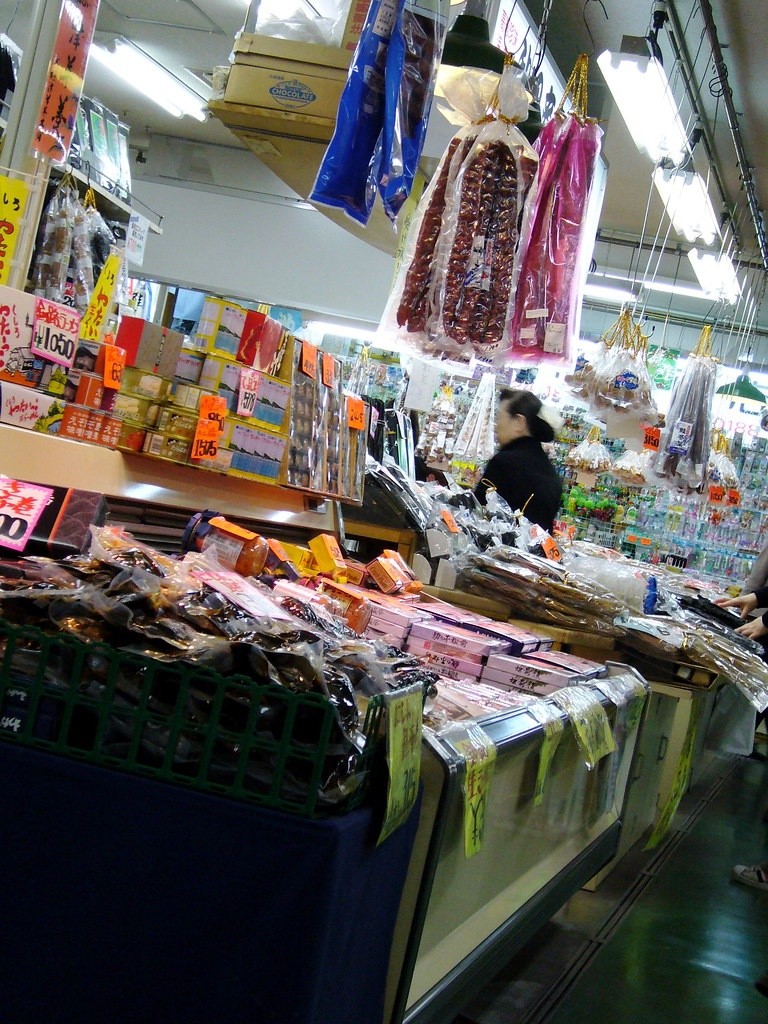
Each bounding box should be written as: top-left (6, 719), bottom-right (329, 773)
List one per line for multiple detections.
top-left (714, 269), bottom-right (766, 406)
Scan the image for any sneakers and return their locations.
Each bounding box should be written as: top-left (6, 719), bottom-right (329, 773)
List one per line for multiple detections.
top-left (732, 865), bottom-right (768, 890)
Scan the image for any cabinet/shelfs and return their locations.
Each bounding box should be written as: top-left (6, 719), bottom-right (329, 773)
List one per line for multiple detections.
top-left (0, 99), bottom-right (165, 325)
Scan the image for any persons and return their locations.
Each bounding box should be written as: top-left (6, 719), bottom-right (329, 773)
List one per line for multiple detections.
top-left (469, 390), bottom-right (563, 535)
top-left (714, 544), bottom-right (768, 887)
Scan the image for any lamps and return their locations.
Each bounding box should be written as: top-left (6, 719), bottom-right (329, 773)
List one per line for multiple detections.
top-left (595, 0), bottom-right (744, 304)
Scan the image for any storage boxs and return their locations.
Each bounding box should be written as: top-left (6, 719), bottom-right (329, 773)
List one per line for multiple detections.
top-left (263, 534), bottom-right (607, 697)
top-left (115, 317), bottom-right (185, 379)
top-left (0, 284), bottom-right (127, 437)
top-left (238, 309), bottom-right (292, 378)
top-left (278, 334), bottom-right (369, 504)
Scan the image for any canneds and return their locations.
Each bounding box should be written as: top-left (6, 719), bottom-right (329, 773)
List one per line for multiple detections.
top-left (184, 510), bottom-right (374, 632)
top-left (57, 370), bottom-right (124, 450)
